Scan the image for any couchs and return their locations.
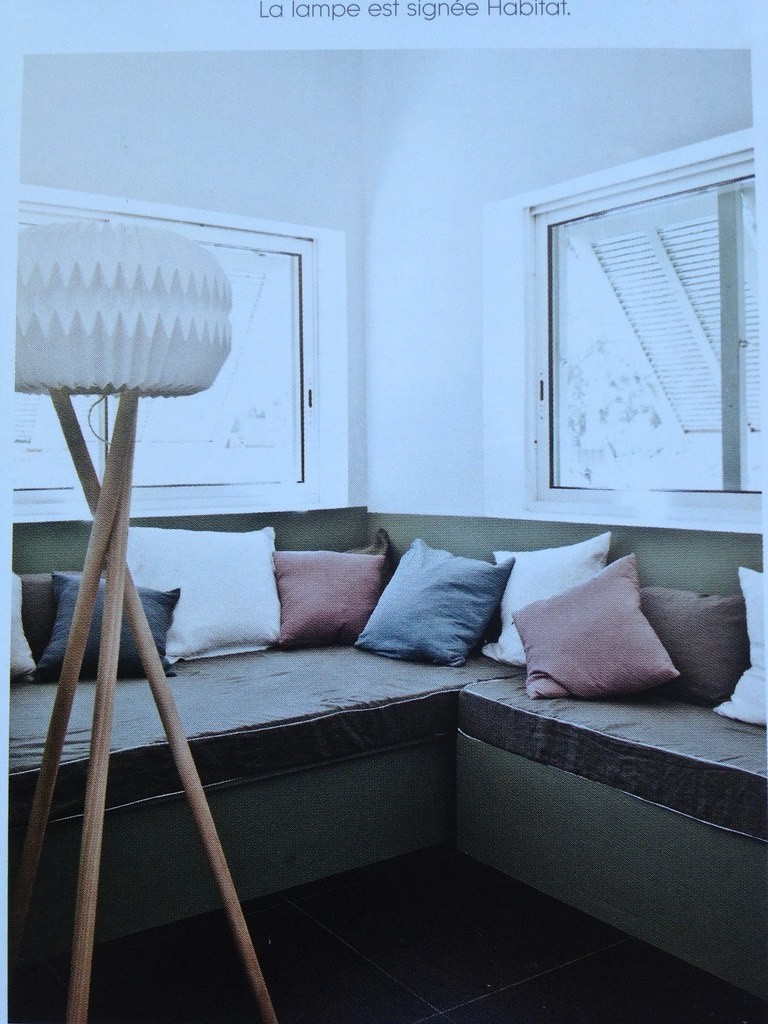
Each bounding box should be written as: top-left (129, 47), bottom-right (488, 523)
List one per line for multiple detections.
top-left (7, 507), bottom-right (768, 1001)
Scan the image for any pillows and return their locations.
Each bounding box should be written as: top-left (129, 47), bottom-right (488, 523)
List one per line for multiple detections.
top-left (272, 551), bottom-right (386, 645)
top-left (713, 566), bottom-right (767, 727)
top-left (15, 574), bottom-right (60, 665)
top-left (640, 585), bottom-right (752, 709)
top-left (31, 571), bottom-right (181, 679)
top-left (119, 527), bottom-right (283, 664)
top-left (11, 572), bottom-right (37, 680)
top-left (512, 551), bottom-right (682, 699)
top-left (353, 536), bottom-right (516, 666)
top-left (480, 531), bottom-right (613, 665)
